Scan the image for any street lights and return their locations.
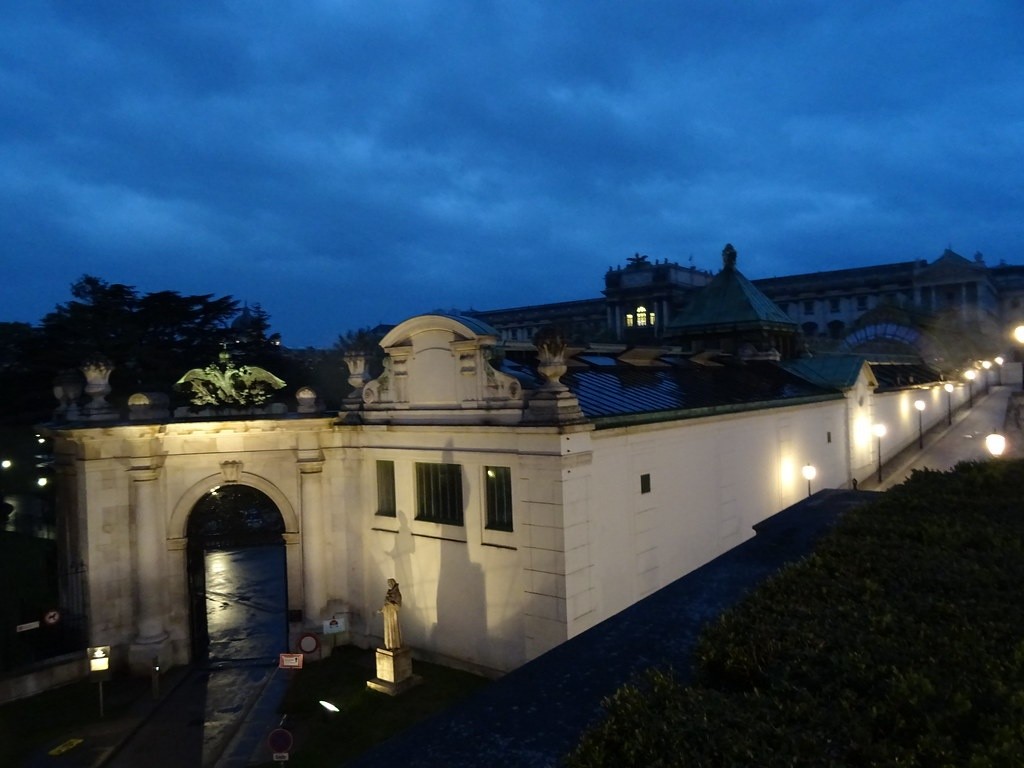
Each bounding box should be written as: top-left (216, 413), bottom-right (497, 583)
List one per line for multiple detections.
top-left (797, 355), bottom-right (1009, 497)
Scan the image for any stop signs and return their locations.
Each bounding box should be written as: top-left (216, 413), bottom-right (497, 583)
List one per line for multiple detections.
top-left (268, 729), bottom-right (295, 753)
